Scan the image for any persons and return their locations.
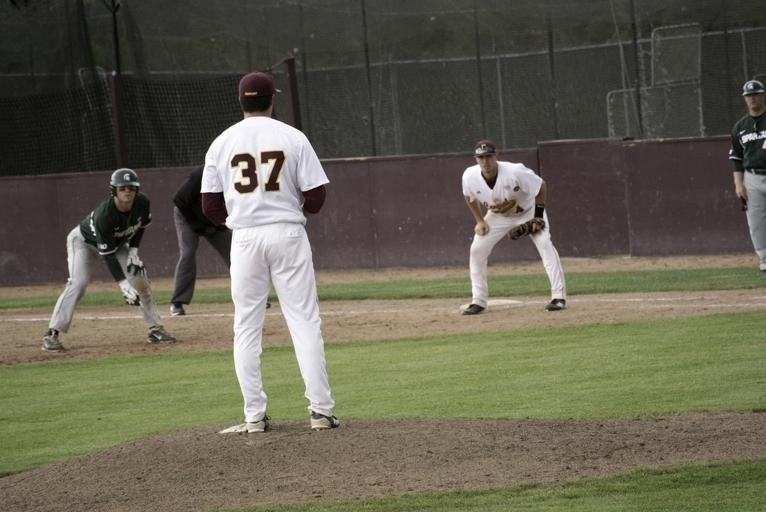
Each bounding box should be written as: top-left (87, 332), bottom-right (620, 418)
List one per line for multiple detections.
top-left (461, 139), bottom-right (567, 316)
top-left (728, 80), bottom-right (766, 271)
top-left (41, 167), bottom-right (176, 353)
top-left (199, 71), bottom-right (339, 434)
top-left (170, 165), bottom-right (271, 317)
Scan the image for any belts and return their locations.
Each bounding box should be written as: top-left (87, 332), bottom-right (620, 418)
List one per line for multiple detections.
top-left (746, 168), bottom-right (766, 175)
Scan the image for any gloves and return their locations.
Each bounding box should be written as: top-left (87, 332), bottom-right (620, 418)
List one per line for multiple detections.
top-left (127, 247), bottom-right (147, 277)
top-left (118, 279), bottom-right (140, 305)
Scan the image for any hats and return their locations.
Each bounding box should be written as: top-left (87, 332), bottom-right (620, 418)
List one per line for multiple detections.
top-left (239, 72), bottom-right (283, 98)
top-left (474, 141), bottom-right (495, 156)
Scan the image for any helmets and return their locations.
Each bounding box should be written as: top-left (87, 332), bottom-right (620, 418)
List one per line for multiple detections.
top-left (111, 168), bottom-right (139, 187)
top-left (742, 80), bottom-right (764, 97)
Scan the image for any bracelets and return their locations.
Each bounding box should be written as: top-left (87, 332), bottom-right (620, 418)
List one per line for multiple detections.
top-left (535, 204), bottom-right (545, 218)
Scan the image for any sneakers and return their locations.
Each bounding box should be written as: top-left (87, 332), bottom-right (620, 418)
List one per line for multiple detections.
top-left (146, 326), bottom-right (176, 344)
top-left (462, 304), bottom-right (485, 316)
top-left (247, 414), bottom-right (270, 433)
top-left (42, 336), bottom-right (63, 353)
top-left (311, 412), bottom-right (340, 431)
top-left (546, 299), bottom-right (565, 311)
top-left (170, 303), bottom-right (184, 315)
